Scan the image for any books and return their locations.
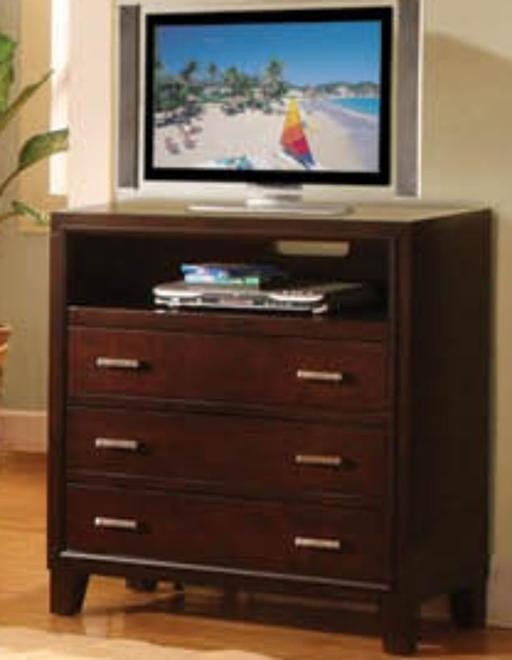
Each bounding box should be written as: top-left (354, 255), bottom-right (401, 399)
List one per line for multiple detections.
top-left (179, 261), bottom-right (281, 287)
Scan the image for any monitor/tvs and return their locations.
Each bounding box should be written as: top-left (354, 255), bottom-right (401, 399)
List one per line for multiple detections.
top-left (112, 0), bottom-right (424, 214)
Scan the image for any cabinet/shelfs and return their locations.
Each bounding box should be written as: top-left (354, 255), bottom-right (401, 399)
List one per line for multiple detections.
top-left (45, 195), bottom-right (489, 654)
